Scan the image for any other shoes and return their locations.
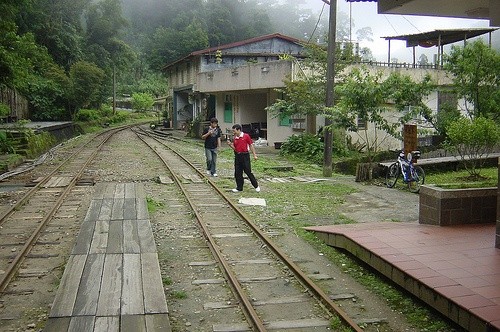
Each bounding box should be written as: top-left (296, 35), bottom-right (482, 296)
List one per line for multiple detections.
top-left (206, 170), bottom-right (210, 175)
top-left (255, 186), bottom-right (260, 192)
top-left (231, 188), bottom-right (243, 192)
top-left (213, 173), bottom-right (218, 177)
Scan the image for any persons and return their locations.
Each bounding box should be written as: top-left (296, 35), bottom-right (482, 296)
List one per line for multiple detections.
top-left (227, 124), bottom-right (260, 193)
top-left (202, 118), bottom-right (222, 177)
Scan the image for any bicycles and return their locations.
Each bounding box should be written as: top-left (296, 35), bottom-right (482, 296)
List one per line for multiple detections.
top-left (386, 149), bottom-right (425, 193)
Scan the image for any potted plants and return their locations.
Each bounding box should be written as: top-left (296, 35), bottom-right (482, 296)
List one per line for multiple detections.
top-left (161, 110), bottom-right (171, 128)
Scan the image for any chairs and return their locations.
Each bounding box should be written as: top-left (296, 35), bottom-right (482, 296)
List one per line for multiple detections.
top-left (241, 123), bottom-right (255, 139)
top-left (261, 121), bottom-right (267, 140)
top-left (251, 122), bottom-right (264, 138)
